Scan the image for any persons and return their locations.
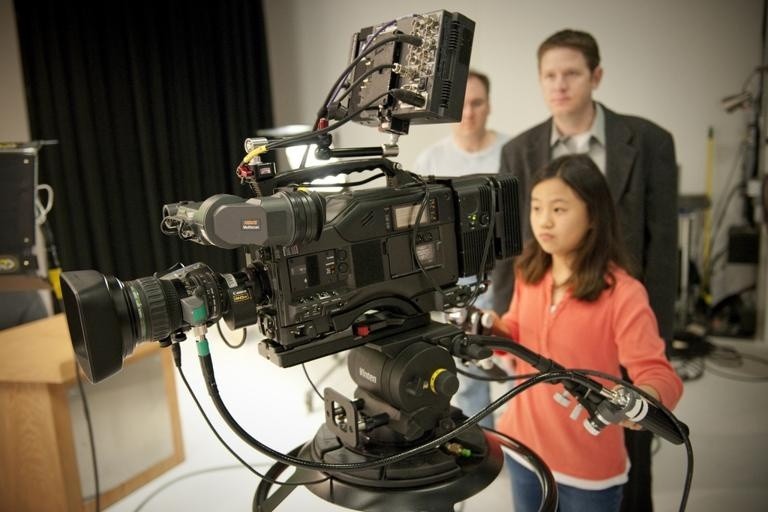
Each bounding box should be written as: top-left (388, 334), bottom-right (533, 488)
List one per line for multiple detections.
top-left (410, 70), bottom-right (514, 437)
top-left (471, 151), bottom-right (681, 512)
top-left (487, 25), bottom-right (679, 511)
